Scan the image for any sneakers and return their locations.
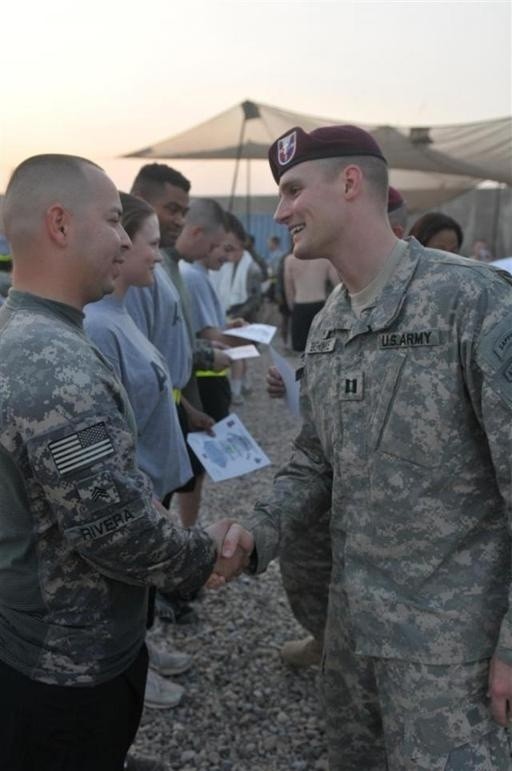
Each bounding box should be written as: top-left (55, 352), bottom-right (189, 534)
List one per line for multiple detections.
top-left (144, 630), bottom-right (191, 709)
top-left (281, 636), bottom-right (321, 665)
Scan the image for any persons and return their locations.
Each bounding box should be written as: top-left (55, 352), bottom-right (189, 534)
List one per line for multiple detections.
top-left (280, 187), bottom-right (409, 668)
top-left (83, 191), bottom-right (194, 707)
top-left (0, 154), bottom-right (250, 771)
top-left (133, 163), bottom-right (342, 624)
top-left (468, 239), bottom-right (495, 261)
top-left (408, 211), bottom-right (463, 254)
top-left (206, 124), bottom-right (512, 771)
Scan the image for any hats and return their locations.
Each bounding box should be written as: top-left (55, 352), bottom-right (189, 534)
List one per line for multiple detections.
top-left (268, 125), bottom-right (387, 185)
